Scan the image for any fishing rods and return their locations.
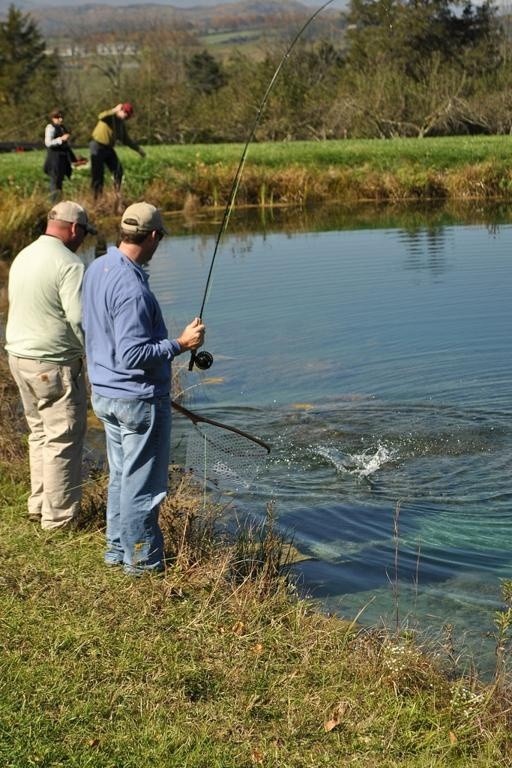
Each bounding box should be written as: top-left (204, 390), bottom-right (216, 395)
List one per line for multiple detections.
top-left (187, 0), bottom-right (360, 370)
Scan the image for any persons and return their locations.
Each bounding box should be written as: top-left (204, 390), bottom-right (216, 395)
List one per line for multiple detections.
top-left (89, 100), bottom-right (147, 203)
top-left (81, 201), bottom-right (205, 580)
top-left (3, 199), bottom-right (98, 535)
top-left (43, 109), bottom-right (79, 203)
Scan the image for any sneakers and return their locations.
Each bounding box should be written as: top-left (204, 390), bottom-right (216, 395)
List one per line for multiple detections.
top-left (28, 513), bottom-right (41, 521)
top-left (48, 109), bottom-right (65, 118)
top-left (48, 199), bottom-right (98, 236)
top-left (121, 201), bottom-right (169, 236)
top-left (122, 102), bottom-right (134, 117)
